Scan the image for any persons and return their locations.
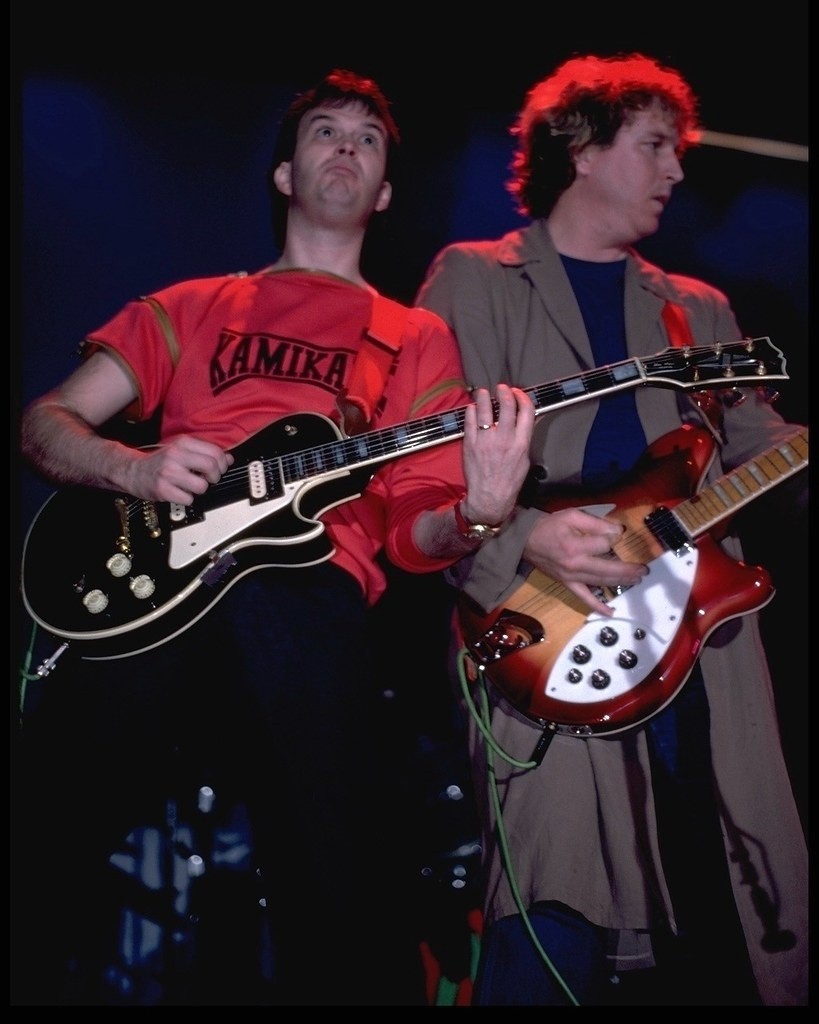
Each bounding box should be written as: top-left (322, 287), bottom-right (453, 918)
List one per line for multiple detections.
top-left (414, 49), bottom-right (808, 1006)
top-left (23, 64), bottom-right (539, 1004)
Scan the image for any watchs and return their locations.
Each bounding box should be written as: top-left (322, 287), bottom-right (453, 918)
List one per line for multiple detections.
top-left (453, 498), bottom-right (502, 543)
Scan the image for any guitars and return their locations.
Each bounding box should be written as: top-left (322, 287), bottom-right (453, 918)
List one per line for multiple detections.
top-left (20, 335), bottom-right (790, 661)
top-left (455, 417), bottom-right (809, 739)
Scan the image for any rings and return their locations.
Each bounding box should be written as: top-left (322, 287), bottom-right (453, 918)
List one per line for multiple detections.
top-left (479, 425), bottom-right (492, 430)
